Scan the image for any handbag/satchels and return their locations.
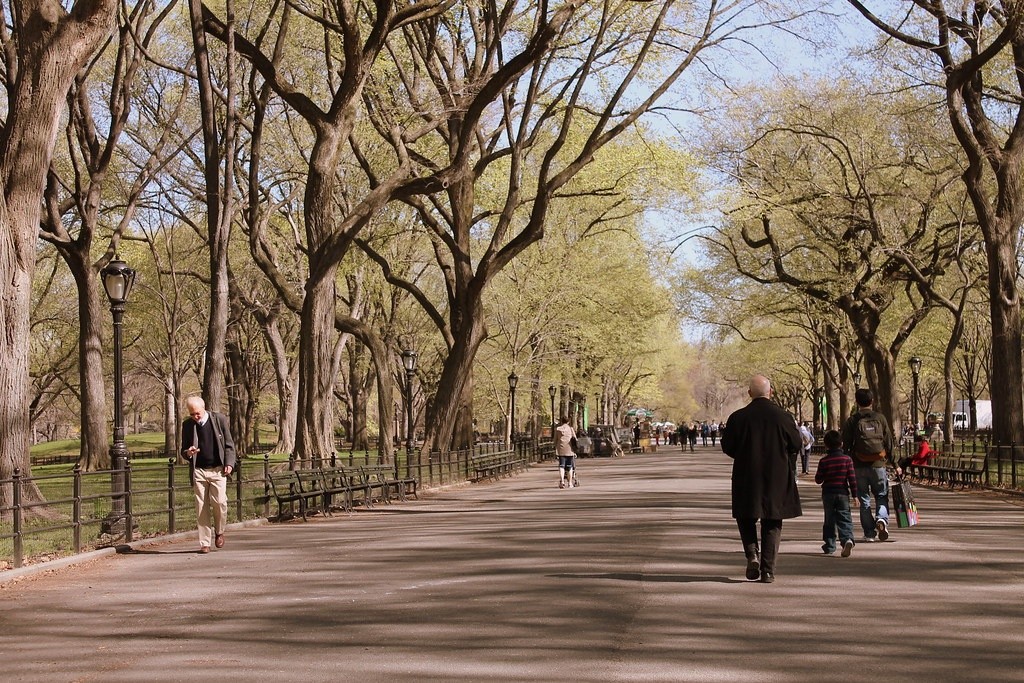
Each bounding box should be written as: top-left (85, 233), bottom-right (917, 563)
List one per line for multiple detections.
top-left (803, 436), bottom-right (809, 446)
top-left (891, 472), bottom-right (919, 528)
top-left (232, 453), bottom-right (241, 474)
top-left (570, 427), bottom-right (578, 450)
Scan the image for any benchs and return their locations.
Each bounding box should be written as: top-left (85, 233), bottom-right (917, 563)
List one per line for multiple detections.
top-left (268, 462), bottom-right (417, 522)
top-left (470, 450), bottom-right (529, 482)
top-left (902, 449), bottom-right (988, 490)
top-left (811, 440), bottom-right (828, 455)
top-left (538, 442), bottom-right (559, 461)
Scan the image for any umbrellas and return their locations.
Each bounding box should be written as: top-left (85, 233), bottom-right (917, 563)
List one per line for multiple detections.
top-left (627, 407), bottom-right (674, 427)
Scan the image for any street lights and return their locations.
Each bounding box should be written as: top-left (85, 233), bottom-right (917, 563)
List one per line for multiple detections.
top-left (99, 259), bottom-right (139, 534)
top-left (820, 386), bottom-right (824, 432)
top-left (796, 394), bottom-right (804, 428)
top-left (507, 369), bottom-right (519, 454)
top-left (594, 393), bottom-right (605, 427)
top-left (908, 357), bottom-right (921, 436)
top-left (548, 386), bottom-right (558, 446)
top-left (398, 349), bottom-right (417, 492)
top-left (852, 371), bottom-right (861, 411)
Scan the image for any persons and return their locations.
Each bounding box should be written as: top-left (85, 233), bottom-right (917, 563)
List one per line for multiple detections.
top-left (842, 388), bottom-right (902, 542)
top-left (813, 429), bottom-right (861, 558)
top-left (900, 436), bottom-right (932, 481)
top-left (553, 415), bottom-right (578, 488)
top-left (631, 418), bottom-right (726, 454)
top-left (721, 375), bottom-right (803, 582)
top-left (794, 419), bottom-right (815, 479)
top-left (180, 397), bottom-right (236, 554)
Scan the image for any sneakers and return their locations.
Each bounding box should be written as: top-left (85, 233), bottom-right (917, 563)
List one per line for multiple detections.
top-left (863, 535), bottom-right (875, 542)
top-left (841, 540), bottom-right (853, 557)
top-left (825, 551), bottom-right (834, 555)
top-left (876, 518), bottom-right (889, 541)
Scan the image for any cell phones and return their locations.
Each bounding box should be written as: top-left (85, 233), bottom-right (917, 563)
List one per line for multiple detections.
top-left (191, 449), bottom-right (196, 451)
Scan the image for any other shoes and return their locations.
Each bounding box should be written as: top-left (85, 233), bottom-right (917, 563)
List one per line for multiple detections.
top-left (893, 477), bottom-right (902, 482)
top-left (568, 484), bottom-right (573, 488)
top-left (802, 470), bottom-right (810, 475)
top-left (560, 484), bottom-right (565, 489)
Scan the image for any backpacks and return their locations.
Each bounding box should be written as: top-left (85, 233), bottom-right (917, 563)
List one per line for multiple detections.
top-left (854, 412), bottom-right (884, 456)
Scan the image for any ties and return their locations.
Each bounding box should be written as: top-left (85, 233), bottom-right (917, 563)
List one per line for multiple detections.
top-left (200, 421), bottom-right (203, 426)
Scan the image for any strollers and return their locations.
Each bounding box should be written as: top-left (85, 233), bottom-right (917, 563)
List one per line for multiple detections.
top-left (557, 450), bottom-right (580, 487)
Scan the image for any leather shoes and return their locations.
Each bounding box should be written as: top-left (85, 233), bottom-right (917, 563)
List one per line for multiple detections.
top-left (746, 554), bottom-right (760, 580)
top-left (761, 572), bottom-right (775, 583)
top-left (214, 531), bottom-right (224, 548)
top-left (201, 546), bottom-right (211, 553)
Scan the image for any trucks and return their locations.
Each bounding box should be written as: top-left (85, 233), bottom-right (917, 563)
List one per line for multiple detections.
top-left (952, 400), bottom-right (993, 429)
